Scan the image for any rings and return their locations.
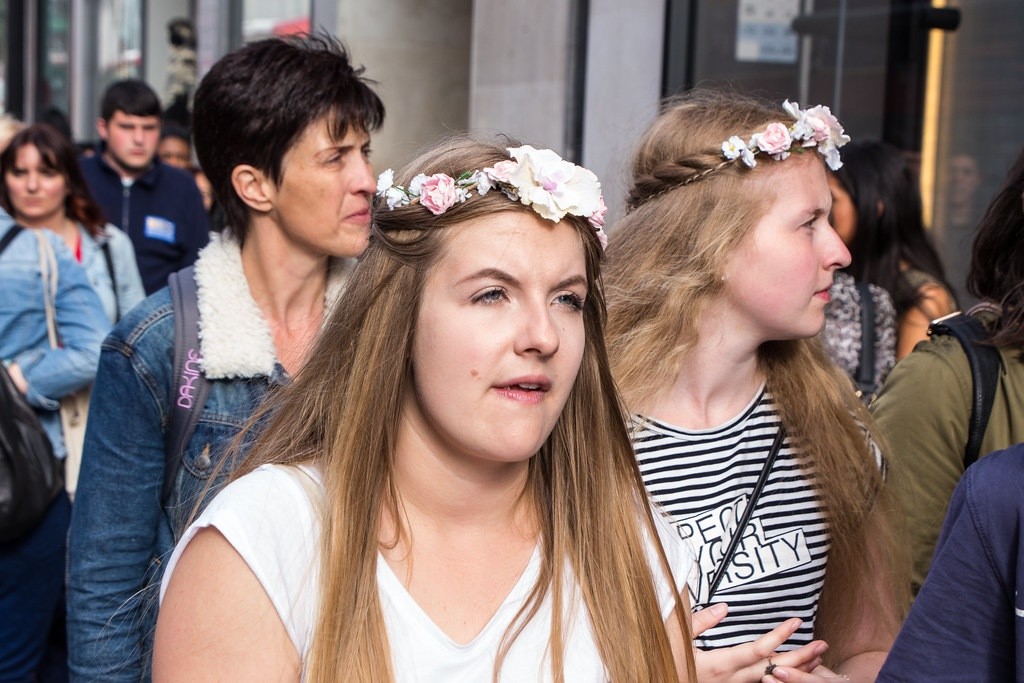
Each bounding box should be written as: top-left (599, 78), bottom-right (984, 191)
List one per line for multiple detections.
top-left (766, 656), bottom-right (776, 674)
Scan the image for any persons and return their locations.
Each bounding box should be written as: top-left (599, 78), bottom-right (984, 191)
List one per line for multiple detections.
top-left (1, 78), bottom-right (213, 682)
top-left (874, 442), bottom-right (1024, 683)
top-left (870, 148), bottom-right (1024, 619)
top-left (947, 157), bottom-right (989, 288)
top-left (820, 140), bottom-right (959, 404)
top-left (151, 137), bottom-right (698, 681)
top-left (65, 21), bottom-right (387, 682)
top-left (604, 88), bottom-right (910, 683)
